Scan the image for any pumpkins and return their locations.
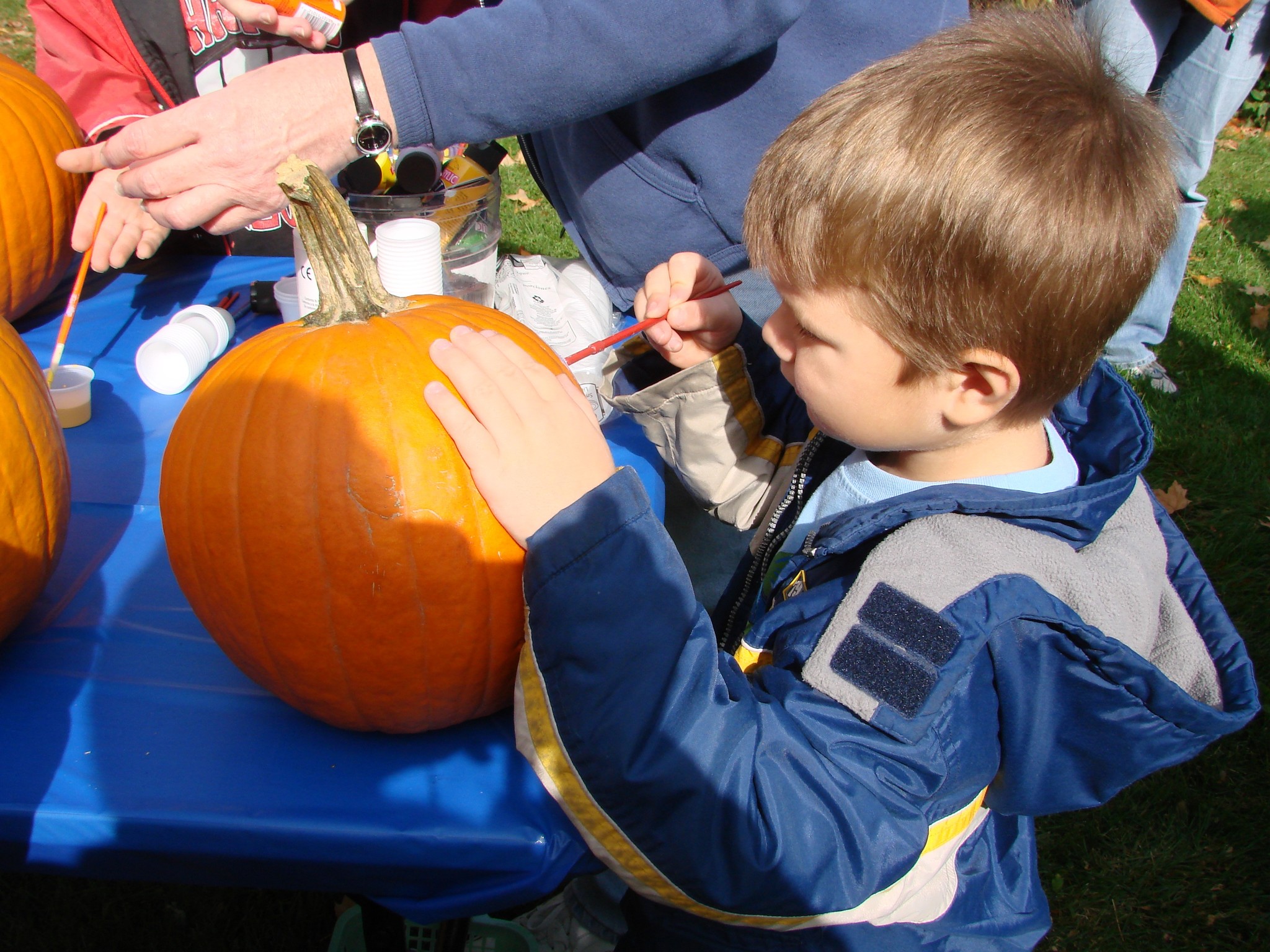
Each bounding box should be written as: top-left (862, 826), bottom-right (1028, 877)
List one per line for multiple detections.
top-left (0, 314), bottom-right (71, 646)
top-left (158, 160), bottom-right (585, 728)
top-left (0, 53), bottom-right (89, 326)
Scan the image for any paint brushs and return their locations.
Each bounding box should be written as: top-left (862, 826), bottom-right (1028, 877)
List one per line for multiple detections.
top-left (47, 203), bottom-right (107, 389)
top-left (563, 281), bottom-right (742, 365)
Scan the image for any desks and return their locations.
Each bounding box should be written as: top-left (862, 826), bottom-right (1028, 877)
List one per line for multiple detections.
top-left (0, 248), bottom-right (678, 952)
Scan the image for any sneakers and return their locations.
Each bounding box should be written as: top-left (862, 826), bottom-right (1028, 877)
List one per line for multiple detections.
top-left (1128, 360), bottom-right (1177, 394)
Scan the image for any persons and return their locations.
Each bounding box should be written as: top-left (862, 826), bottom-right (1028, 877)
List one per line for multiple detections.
top-left (423, 0), bottom-right (1259, 952)
top-left (24, 0), bottom-right (502, 273)
top-left (1083, 0), bottom-right (1270, 394)
top-left (103, 0), bottom-right (970, 329)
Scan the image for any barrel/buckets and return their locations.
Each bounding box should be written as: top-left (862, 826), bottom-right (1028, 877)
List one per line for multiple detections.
top-left (347, 164), bottom-right (502, 309)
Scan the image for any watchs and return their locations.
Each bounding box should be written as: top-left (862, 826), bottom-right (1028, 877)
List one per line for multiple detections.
top-left (343, 48), bottom-right (392, 158)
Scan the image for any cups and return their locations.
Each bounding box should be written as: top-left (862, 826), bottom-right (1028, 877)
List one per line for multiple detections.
top-left (293, 219), bottom-right (368, 318)
top-left (273, 276), bottom-right (300, 321)
top-left (135, 305), bottom-right (235, 395)
top-left (375, 218), bottom-right (443, 297)
top-left (43, 365), bottom-right (95, 429)
top-left (548, 259), bottom-right (609, 386)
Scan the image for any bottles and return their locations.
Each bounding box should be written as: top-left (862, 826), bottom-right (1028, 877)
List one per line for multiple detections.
top-left (337, 148), bottom-right (399, 196)
top-left (412, 139), bottom-right (508, 254)
top-left (396, 142), bottom-right (444, 194)
top-left (245, 0), bottom-right (346, 42)
